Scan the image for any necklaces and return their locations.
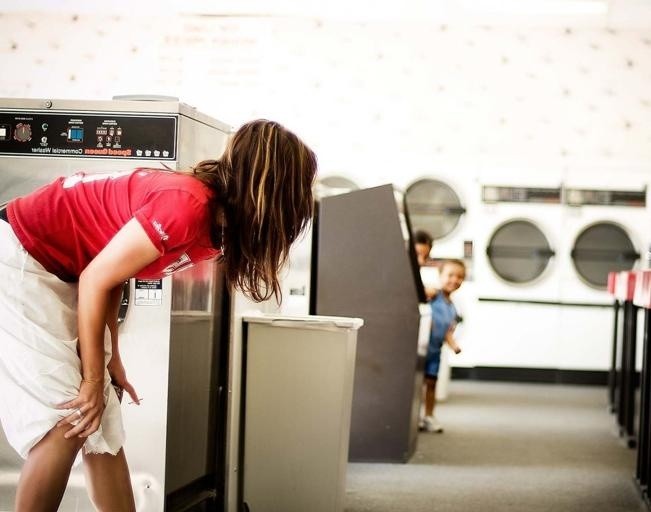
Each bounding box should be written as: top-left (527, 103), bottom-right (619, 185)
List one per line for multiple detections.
top-left (218, 207), bottom-right (227, 259)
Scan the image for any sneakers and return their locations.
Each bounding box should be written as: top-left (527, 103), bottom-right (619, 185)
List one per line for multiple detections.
top-left (419, 416), bottom-right (444, 434)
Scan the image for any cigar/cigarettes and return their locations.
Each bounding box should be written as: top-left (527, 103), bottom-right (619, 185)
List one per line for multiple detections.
top-left (126, 397), bottom-right (145, 405)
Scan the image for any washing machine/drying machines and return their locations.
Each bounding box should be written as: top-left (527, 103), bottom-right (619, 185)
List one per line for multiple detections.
top-left (1, 99), bottom-right (650, 512)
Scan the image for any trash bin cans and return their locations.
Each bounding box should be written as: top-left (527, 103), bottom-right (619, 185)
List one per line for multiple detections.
top-left (241, 313), bottom-right (363, 512)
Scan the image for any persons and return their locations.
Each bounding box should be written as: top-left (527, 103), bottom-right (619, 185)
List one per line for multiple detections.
top-left (413, 229), bottom-right (440, 301)
top-left (0, 116), bottom-right (319, 511)
top-left (416, 256), bottom-right (467, 434)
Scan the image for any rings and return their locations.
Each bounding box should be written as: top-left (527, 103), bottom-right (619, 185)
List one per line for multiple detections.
top-left (76, 409), bottom-right (84, 419)
